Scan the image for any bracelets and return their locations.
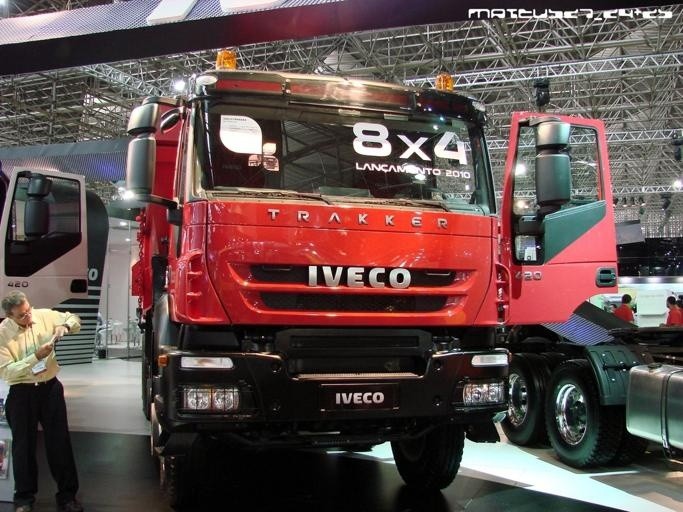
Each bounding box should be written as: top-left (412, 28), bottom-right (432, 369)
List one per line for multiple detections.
top-left (61, 322), bottom-right (71, 332)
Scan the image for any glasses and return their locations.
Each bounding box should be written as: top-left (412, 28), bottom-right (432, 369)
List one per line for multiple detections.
top-left (9, 306), bottom-right (33, 322)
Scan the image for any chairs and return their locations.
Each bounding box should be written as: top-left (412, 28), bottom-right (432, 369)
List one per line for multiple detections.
top-left (111, 323), bottom-right (124, 344)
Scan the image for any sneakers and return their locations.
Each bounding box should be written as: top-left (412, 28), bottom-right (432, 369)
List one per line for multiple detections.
top-left (59, 498), bottom-right (84, 512)
top-left (15, 503), bottom-right (32, 512)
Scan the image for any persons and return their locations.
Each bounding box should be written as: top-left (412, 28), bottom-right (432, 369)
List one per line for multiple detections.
top-left (674, 300), bottom-right (682, 326)
top-left (658, 295), bottom-right (680, 328)
top-left (613, 294), bottom-right (634, 325)
top-left (0, 288), bottom-right (85, 512)
